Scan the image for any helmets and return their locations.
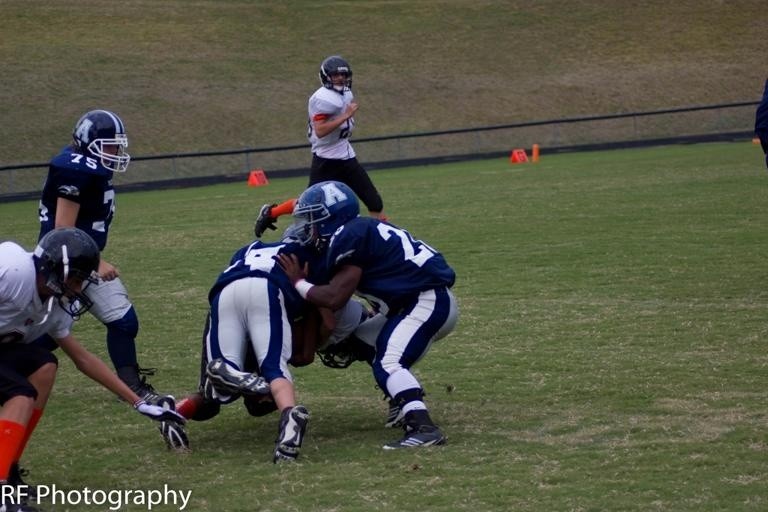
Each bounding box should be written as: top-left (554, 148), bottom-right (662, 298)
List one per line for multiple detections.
top-left (291, 179), bottom-right (361, 249)
top-left (318, 56), bottom-right (352, 92)
top-left (71, 109), bottom-right (131, 173)
top-left (314, 336), bottom-right (367, 369)
top-left (280, 223), bottom-right (318, 246)
top-left (32, 227), bottom-right (100, 321)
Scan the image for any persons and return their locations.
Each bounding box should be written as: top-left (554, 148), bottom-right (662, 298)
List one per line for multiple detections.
top-left (254, 55), bottom-right (387, 239)
top-left (35, 108), bottom-right (163, 404)
top-left (158, 180), bottom-right (461, 464)
top-left (1, 226), bottom-right (187, 512)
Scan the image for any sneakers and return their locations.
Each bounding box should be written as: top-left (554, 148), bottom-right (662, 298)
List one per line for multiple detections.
top-left (117, 376), bottom-right (176, 406)
top-left (7, 462), bottom-right (39, 500)
top-left (273, 404), bottom-right (309, 464)
top-left (254, 203), bottom-right (278, 238)
top-left (1, 483), bottom-right (37, 512)
top-left (381, 423), bottom-right (449, 450)
top-left (384, 398), bottom-right (407, 429)
top-left (205, 358), bottom-right (271, 397)
top-left (157, 394), bottom-right (190, 452)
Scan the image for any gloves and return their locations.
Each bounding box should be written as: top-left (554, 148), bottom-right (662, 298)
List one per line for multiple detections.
top-left (137, 403), bottom-right (187, 425)
